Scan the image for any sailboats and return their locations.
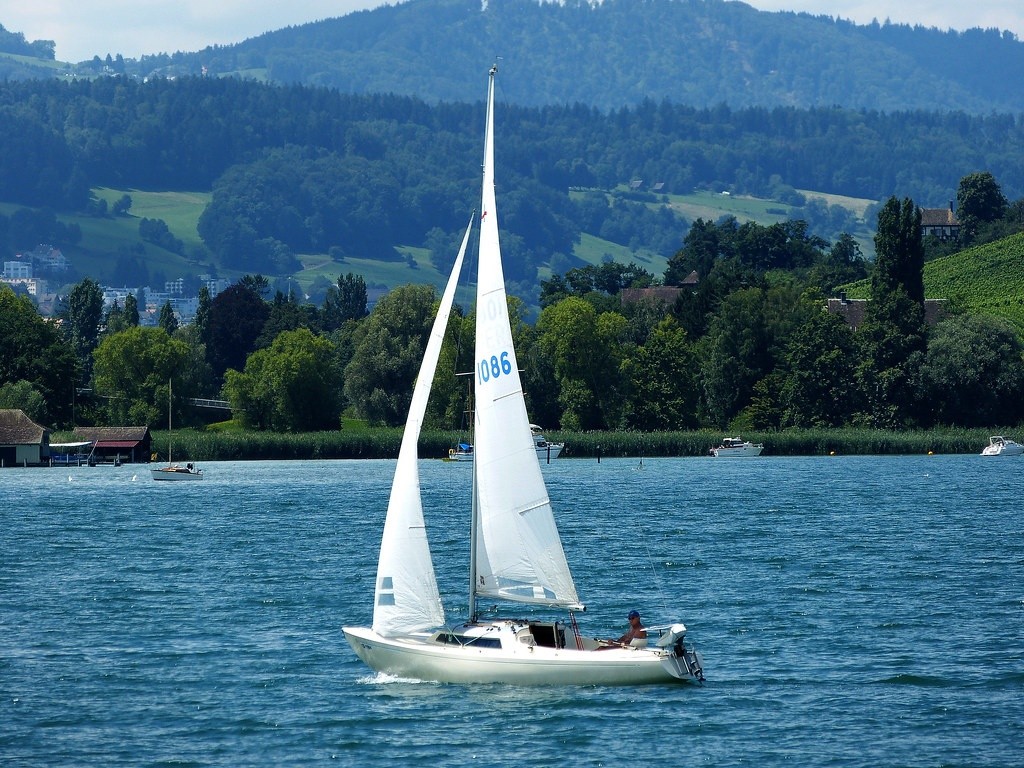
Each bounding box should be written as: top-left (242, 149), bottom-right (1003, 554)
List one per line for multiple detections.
top-left (149, 377), bottom-right (204, 481)
top-left (343, 67), bottom-right (712, 687)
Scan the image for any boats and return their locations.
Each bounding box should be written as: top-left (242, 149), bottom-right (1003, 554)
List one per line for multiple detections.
top-left (441, 368), bottom-right (565, 462)
top-left (709, 435), bottom-right (765, 457)
top-left (979, 435), bottom-right (1024, 456)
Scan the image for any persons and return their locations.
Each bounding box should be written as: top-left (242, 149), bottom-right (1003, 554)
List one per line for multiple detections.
top-left (594, 610), bottom-right (647, 651)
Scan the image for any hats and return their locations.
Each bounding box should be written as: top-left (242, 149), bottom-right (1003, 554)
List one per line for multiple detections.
top-left (627, 610), bottom-right (639, 617)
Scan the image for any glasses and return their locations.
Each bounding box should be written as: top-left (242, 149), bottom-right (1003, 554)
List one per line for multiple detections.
top-left (628, 617), bottom-right (634, 620)
